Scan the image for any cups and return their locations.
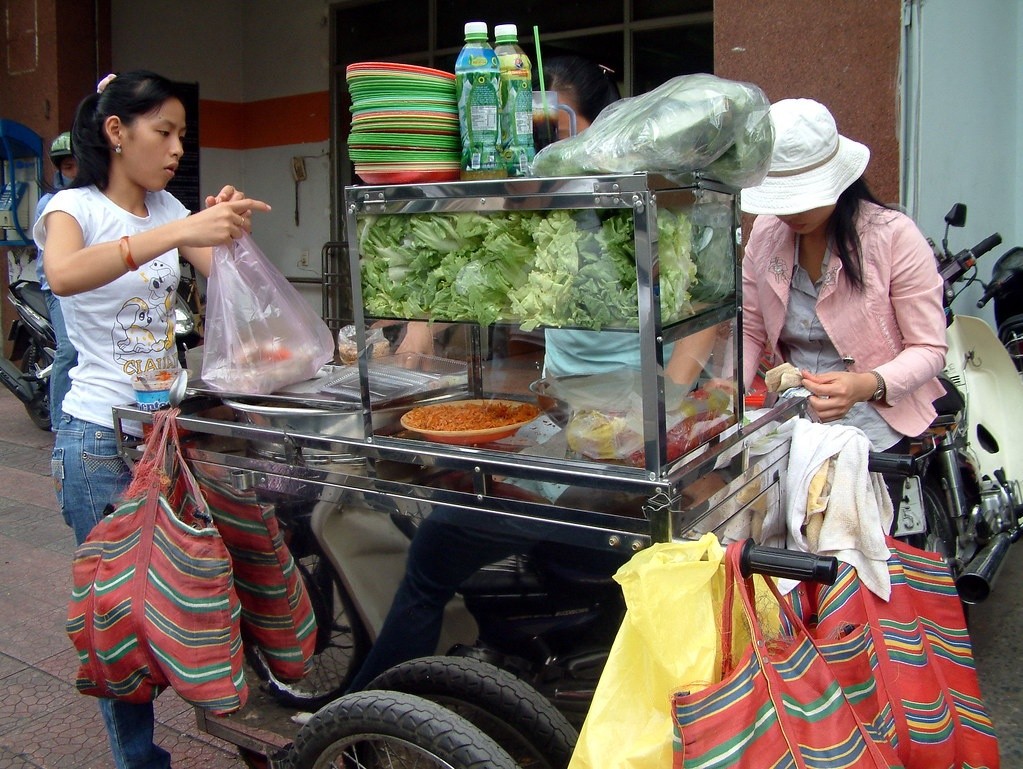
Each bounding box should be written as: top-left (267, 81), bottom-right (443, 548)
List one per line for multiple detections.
top-left (529, 89), bottom-right (578, 155)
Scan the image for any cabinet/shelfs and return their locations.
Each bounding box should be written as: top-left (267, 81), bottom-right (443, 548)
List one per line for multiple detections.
top-left (343, 171), bottom-right (745, 476)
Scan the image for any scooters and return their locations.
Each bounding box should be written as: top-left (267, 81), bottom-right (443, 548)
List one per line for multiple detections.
top-left (875, 203), bottom-right (1023, 629)
top-left (1, 269), bottom-right (204, 437)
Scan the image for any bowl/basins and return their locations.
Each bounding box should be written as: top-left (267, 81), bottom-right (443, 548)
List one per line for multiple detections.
top-left (132, 369), bottom-right (192, 410)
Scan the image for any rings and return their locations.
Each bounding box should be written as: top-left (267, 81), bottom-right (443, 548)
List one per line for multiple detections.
top-left (241, 216), bottom-right (245, 227)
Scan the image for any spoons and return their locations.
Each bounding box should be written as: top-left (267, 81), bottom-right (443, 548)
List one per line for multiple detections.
top-left (169, 371), bottom-right (188, 409)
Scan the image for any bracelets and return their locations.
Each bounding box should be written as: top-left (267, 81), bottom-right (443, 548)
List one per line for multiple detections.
top-left (119, 236), bottom-right (138, 271)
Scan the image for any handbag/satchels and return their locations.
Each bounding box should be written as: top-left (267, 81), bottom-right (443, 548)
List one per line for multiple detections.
top-left (64, 407), bottom-right (318, 716)
top-left (199, 229), bottom-right (336, 397)
top-left (558, 527), bottom-right (1003, 769)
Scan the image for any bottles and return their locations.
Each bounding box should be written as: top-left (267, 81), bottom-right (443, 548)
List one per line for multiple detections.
top-left (455, 22), bottom-right (507, 181)
top-left (493, 24), bottom-right (534, 178)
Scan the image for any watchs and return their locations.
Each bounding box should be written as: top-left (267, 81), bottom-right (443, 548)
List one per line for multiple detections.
top-left (869, 372), bottom-right (886, 403)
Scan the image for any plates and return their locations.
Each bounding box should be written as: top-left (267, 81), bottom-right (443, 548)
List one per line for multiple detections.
top-left (346, 62), bottom-right (459, 184)
top-left (400, 399), bottom-right (541, 444)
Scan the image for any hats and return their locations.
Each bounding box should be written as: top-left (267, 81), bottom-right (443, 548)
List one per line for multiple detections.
top-left (740, 98), bottom-right (870, 215)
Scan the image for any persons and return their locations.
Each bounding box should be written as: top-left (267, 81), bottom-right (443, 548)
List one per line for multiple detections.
top-left (721, 98), bottom-right (946, 505)
top-left (330, 483), bottom-right (669, 705)
top-left (33, 70), bottom-right (272, 769)
top-left (374, 58), bottom-right (725, 404)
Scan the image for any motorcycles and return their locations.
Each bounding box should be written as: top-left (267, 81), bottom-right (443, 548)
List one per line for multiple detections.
top-left (241, 502), bottom-right (629, 718)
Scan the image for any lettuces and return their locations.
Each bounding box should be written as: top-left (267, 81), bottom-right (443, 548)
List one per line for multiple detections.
top-left (359, 205), bottom-right (702, 332)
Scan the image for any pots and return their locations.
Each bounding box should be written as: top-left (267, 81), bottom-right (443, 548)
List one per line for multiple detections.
top-left (216, 389), bottom-right (475, 456)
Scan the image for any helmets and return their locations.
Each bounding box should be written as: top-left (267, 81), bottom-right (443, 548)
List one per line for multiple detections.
top-left (49, 131), bottom-right (73, 165)
